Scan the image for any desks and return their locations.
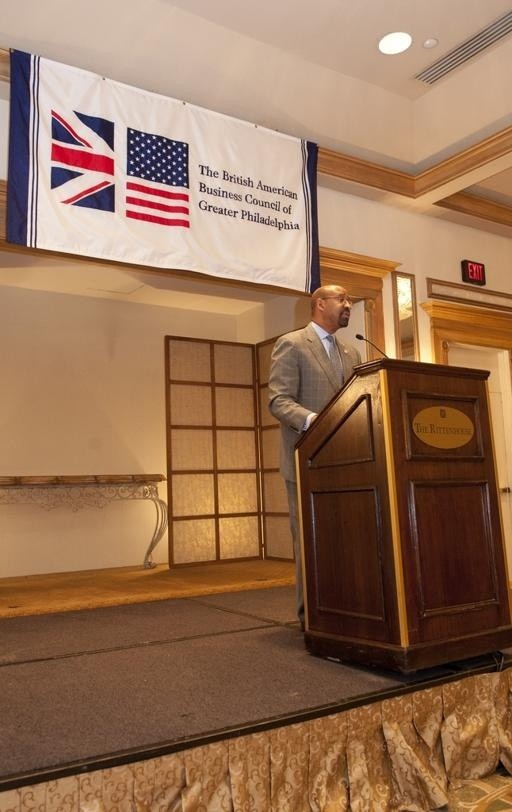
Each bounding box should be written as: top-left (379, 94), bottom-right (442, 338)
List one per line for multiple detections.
top-left (0, 474), bottom-right (167, 569)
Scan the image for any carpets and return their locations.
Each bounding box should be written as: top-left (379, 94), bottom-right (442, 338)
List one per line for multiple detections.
top-left (0, 586), bottom-right (512, 794)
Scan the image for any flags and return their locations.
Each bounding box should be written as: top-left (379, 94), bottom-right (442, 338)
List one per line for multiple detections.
top-left (6, 48), bottom-right (321, 295)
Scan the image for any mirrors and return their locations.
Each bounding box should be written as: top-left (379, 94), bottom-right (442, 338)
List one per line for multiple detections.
top-left (391, 270), bottom-right (420, 362)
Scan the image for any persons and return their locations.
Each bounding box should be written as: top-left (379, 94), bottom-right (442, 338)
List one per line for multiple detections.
top-left (267, 285), bottom-right (361, 631)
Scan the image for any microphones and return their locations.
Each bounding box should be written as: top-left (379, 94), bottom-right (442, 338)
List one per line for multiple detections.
top-left (356, 335), bottom-right (388, 359)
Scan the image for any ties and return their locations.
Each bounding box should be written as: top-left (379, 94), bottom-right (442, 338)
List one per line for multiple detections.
top-left (328, 336), bottom-right (344, 384)
